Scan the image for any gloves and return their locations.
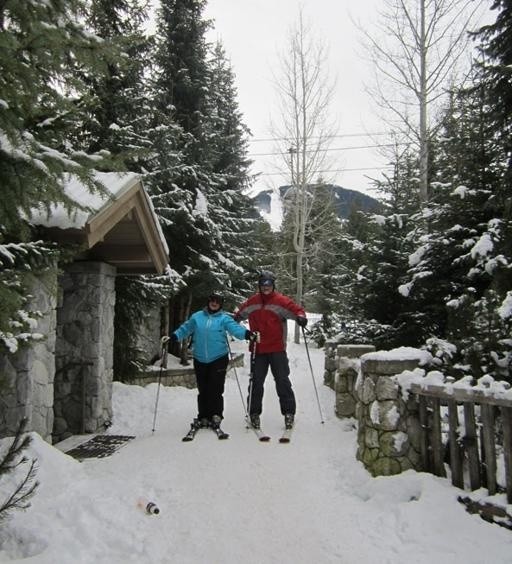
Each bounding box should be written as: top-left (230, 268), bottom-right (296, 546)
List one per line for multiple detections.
top-left (247, 330), bottom-right (260, 343)
top-left (160, 336), bottom-right (172, 343)
top-left (297, 317), bottom-right (308, 328)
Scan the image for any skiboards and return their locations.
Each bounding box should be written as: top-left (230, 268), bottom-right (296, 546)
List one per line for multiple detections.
top-left (182, 417), bottom-right (295, 443)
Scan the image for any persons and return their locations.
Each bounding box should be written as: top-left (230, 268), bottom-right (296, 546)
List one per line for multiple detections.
top-left (162, 290), bottom-right (257, 428)
top-left (233, 273), bottom-right (308, 430)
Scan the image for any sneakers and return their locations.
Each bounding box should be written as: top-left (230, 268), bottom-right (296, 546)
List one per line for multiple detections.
top-left (285, 413), bottom-right (293, 425)
top-left (251, 415), bottom-right (260, 428)
top-left (195, 418), bottom-right (205, 428)
top-left (212, 415), bottom-right (222, 424)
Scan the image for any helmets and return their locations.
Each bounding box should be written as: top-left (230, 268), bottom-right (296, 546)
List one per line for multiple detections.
top-left (209, 290), bottom-right (226, 305)
top-left (258, 279), bottom-right (275, 286)
